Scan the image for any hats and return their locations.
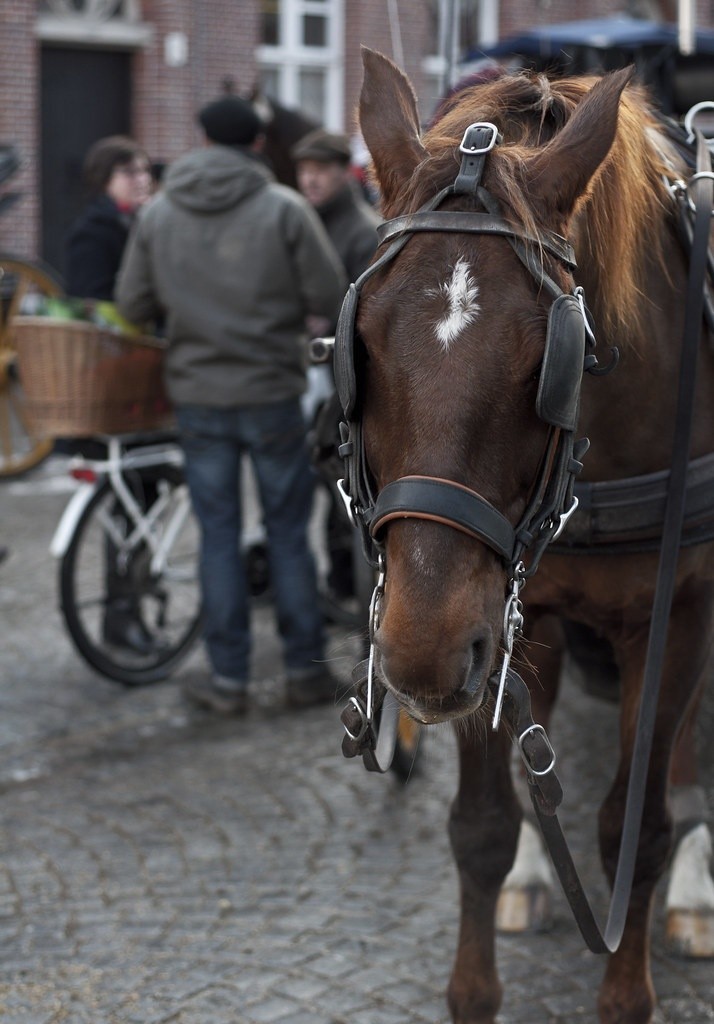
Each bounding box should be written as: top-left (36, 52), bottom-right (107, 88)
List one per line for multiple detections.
top-left (291, 130), bottom-right (351, 160)
top-left (198, 98), bottom-right (259, 145)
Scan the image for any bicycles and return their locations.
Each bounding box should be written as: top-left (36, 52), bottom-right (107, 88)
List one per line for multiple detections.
top-left (52, 365), bottom-right (363, 685)
top-left (0, 139), bottom-right (75, 479)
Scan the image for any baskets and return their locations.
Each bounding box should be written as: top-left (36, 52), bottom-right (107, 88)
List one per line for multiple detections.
top-left (13, 314), bottom-right (167, 440)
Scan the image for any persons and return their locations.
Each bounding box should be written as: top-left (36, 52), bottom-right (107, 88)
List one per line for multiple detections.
top-left (287, 128), bottom-right (394, 624)
top-left (52, 134), bottom-right (157, 327)
top-left (112, 93), bottom-right (355, 717)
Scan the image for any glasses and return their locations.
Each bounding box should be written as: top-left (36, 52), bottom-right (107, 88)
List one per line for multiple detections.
top-left (113, 167), bottom-right (147, 180)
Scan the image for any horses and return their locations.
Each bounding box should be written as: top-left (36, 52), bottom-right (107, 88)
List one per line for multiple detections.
top-left (342, 45), bottom-right (711, 1022)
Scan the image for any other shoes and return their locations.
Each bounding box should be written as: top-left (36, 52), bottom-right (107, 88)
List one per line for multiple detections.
top-left (104, 614), bottom-right (168, 657)
top-left (286, 673), bottom-right (354, 708)
top-left (184, 673), bottom-right (246, 717)
